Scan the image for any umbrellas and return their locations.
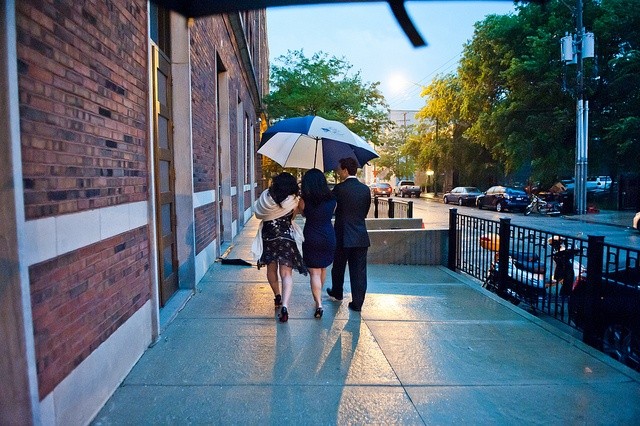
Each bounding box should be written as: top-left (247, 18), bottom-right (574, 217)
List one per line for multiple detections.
top-left (256, 114), bottom-right (381, 174)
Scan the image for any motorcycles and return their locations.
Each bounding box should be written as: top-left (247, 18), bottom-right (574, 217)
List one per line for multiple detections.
top-left (480, 232), bottom-right (588, 288)
top-left (481, 230), bottom-right (588, 311)
top-left (524, 192), bottom-right (563, 217)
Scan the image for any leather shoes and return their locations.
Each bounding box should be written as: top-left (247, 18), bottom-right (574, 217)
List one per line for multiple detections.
top-left (326, 287), bottom-right (343, 302)
top-left (348, 302), bottom-right (361, 311)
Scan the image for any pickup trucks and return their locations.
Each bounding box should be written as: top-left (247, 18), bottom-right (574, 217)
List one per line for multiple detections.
top-left (568, 267), bottom-right (640, 365)
top-left (394, 179), bottom-right (422, 198)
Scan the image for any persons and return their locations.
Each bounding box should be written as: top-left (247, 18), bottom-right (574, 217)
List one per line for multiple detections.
top-left (297, 166), bottom-right (338, 319)
top-left (326, 157), bottom-right (373, 314)
top-left (252, 171), bottom-right (309, 322)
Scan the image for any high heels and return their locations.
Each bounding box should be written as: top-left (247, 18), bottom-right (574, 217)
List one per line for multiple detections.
top-left (314, 308), bottom-right (323, 319)
top-left (278, 306), bottom-right (288, 324)
top-left (274, 296), bottom-right (282, 309)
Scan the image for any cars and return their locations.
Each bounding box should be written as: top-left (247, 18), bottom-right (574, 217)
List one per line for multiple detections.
top-left (443, 186), bottom-right (482, 206)
top-left (370, 182), bottom-right (392, 197)
top-left (476, 185), bottom-right (530, 211)
top-left (632, 211), bottom-right (640, 232)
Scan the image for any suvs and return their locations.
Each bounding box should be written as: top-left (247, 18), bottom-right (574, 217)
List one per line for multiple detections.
top-left (561, 175), bottom-right (613, 194)
